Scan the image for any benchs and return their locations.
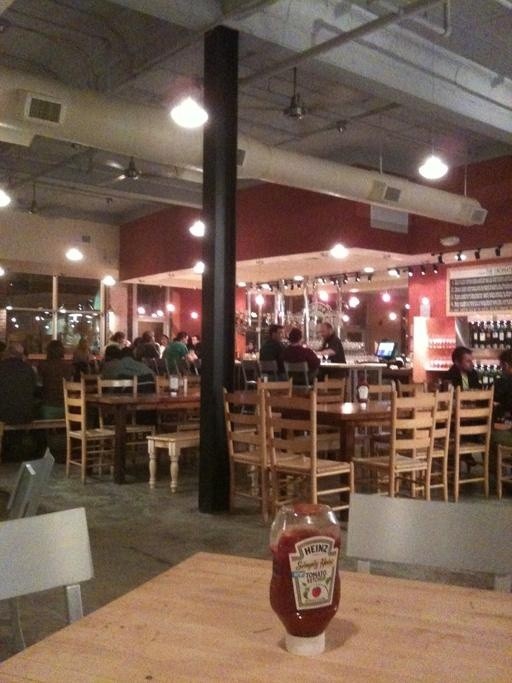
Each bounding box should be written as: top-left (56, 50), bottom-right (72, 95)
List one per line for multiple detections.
top-left (0, 418), bottom-right (65, 447)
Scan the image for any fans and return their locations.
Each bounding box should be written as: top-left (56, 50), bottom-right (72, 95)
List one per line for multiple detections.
top-left (71, 148), bottom-right (175, 190)
top-left (249, 67), bottom-right (347, 134)
top-left (18, 182), bottom-right (48, 216)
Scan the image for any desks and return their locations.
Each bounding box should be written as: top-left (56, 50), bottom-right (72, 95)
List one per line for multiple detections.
top-left (1, 551), bottom-right (512, 683)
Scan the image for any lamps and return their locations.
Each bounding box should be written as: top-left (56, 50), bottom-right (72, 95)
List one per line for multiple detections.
top-left (418, 127), bottom-right (449, 182)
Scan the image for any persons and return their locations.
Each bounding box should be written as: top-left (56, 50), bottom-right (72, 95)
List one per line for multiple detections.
top-left (445, 347), bottom-right (485, 479)
top-left (0, 331), bottom-right (203, 467)
top-left (488, 350), bottom-right (512, 495)
top-left (278, 328), bottom-right (321, 385)
top-left (260, 325), bottom-right (286, 361)
top-left (314, 322), bottom-right (347, 381)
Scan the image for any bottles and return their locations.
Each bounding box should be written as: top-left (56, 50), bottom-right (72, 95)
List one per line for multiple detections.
top-left (356, 383), bottom-right (369, 402)
top-left (472, 320), bottom-right (512, 348)
top-left (270, 502), bottom-right (341, 656)
top-left (168, 375), bottom-right (187, 397)
top-left (476, 364), bottom-right (505, 389)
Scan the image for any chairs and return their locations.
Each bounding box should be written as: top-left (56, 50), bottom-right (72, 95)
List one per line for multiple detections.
top-left (63, 371), bottom-right (512, 523)
top-left (1, 506), bottom-right (95, 624)
top-left (1, 447), bottom-right (57, 512)
